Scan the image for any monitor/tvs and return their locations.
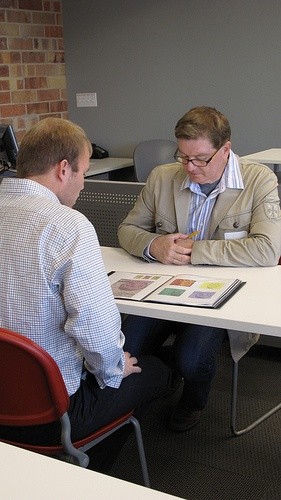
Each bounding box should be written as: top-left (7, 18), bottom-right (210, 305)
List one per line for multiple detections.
top-left (0, 124), bottom-right (20, 175)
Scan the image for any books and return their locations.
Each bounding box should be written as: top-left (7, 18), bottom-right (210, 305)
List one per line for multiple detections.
top-left (107, 270), bottom-right (246, 309)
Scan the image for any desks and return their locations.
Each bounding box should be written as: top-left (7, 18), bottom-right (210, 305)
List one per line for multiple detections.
top-left (0, 443), bottom-right (186, 500)
top-left (102, 247), bottom-right (281, 436)
top-left (84, 157), bottom-right (133, 182)
top-left (239, 147), bottom-right (281, 172)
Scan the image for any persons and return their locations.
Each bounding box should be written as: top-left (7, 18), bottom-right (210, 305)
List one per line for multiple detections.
top-left (0, 116), bottom-right (186, 470)
top-left (117, 104), bottom-right (281, 431)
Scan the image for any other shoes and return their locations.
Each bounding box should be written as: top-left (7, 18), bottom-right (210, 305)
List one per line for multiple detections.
top-left (166, 405), bottom-right (202, 434)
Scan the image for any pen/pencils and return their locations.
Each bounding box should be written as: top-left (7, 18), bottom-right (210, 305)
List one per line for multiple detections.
top-left (187, 229), bottom-right (200, 239)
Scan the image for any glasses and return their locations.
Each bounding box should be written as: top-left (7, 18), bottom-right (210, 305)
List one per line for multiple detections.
top-left (173, 144), bottom-right (223, 167)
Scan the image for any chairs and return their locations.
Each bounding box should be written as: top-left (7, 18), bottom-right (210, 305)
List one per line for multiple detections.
top-left (0, 328), bottom-right (148, 487)
top-left (134, 138), bottom-right (177, 182)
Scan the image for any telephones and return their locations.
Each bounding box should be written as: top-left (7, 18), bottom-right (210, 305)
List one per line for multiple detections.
top-left (90, 143), bottom-right (108, 159)
